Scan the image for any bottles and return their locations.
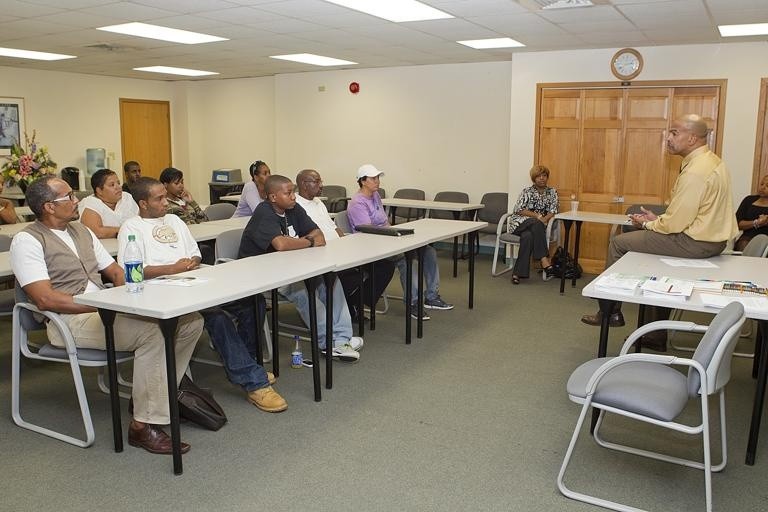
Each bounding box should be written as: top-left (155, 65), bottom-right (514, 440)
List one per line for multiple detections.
top-left (291, 335), bottom-right (303, 369)
top-left (124, 235), bottom-right (144, 293)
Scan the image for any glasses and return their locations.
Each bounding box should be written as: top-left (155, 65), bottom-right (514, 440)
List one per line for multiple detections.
top-left (304, 179), bottom-right (323, 184)
top-left (42, 191), bottom-right (74, 206)
top-left (253, 160), bottom-right (262, 174)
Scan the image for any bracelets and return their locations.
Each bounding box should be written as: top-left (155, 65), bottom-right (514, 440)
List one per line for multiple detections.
top-left (538, 214), bottom-right (542, 220)
top-left (1, 206), bottom-right (5, 211)
top-left (753, 219), bottom-right (759, 230)
top-left (641, 221), bottom-right (646, 230)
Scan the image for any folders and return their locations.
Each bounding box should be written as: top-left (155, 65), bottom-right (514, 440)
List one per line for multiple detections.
top-left (355, 225), bottom-right (415, 236)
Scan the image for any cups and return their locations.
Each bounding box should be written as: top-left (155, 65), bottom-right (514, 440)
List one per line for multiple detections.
top-left (570, 201), bottom-right (580, 217)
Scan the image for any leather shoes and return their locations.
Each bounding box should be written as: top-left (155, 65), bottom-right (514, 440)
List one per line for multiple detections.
top-left (266, 371), bottom-right (276, 385)
top-left (540, 262), bottom-right (552, 273)
top-left (511, 275), bottom-right (519, 284)
top-left (581, 309), bottom-right (624, 328)
top-left (624, 334), bottom-right (667, 352)
top-left (128, 422), bottom-right (191, 455)
top-left (246, 385), bottom-right (288, 413)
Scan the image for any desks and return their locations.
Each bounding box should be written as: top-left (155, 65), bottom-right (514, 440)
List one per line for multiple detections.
top-left (72, 217), bottom-right (488, 476)
top-left (554, 210), bottom-right (635, 298)
top-left (581, 251), bottom-right (767, 466)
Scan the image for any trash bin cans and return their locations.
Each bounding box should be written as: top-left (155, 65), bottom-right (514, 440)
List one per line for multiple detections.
top-left (208, 168), bottom-right (245, 207)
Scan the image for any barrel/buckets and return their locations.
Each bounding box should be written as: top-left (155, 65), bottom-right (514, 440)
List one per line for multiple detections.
top-left (87, 149), bottom-right (106, 175)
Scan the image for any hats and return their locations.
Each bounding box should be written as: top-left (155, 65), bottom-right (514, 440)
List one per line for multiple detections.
top-left (356, 164), bottom-right (384, 181)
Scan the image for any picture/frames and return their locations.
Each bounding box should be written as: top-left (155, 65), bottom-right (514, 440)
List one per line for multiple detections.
top-left (0, 96), bottom-right (27, 155)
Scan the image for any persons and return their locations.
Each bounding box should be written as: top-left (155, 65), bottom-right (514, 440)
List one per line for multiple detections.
top-left (121, 162), bottom-right (141, 193)
top-left (0, 172), bottom-right (22, 224)
top-left (116, 177), bottom-right (288, 412)
top-left (230, 161), bottom-right (272, 218)
top-left (508, 165), bottom-right (560, 284)
top-left (9, 176), bottom-right (204, 454)
top-left (347, 164), bottom-right (455, 321)
top-left (160, 168), bottom-right (216, 266)
top-left (294, 169), bottom-right (364, 320)
top-left (78, 169), bottom-right (140, 239)
top-left (235, 176), bottom-right (363, 362)
top-left (732, 174), bottom-right (768, 257)
top-left (581, 113), bottom-right (739, 352)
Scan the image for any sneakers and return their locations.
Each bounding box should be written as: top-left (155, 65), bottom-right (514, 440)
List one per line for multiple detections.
top-left (424, 295), bottom-right (454, 310)
top-left (410, 304), bottom-right (431, 321)
top-left (321, 336), bottom-right (363, 355)
top-left (331, 345), bottom-right (360, 363)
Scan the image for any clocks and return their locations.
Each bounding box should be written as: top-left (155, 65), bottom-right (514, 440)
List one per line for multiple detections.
top-left (611, 47), bottom-right (643, 80)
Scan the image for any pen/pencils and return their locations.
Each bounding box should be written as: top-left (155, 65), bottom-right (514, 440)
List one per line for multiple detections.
top-left (667, 284), bottom-right (673, 293)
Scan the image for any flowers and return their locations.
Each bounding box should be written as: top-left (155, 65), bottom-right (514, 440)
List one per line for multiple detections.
top-left (0, 129), bottom-right (58, 193)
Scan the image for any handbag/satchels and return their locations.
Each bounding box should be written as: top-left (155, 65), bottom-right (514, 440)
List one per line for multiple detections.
top-left (543, 246), bottom-right (582, 279)
top-left (177, 374), bottom-right (228, 431)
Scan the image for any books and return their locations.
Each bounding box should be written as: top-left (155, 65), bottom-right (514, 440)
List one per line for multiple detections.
top-left (595, 272), bottom-right (724, 306)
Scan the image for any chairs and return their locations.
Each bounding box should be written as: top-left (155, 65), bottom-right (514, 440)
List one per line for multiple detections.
top-left (622, 204), bottom-right (668, 234)
top-left (213, 227), bottom-right (313, 363)
top-left (555, 301), bottom-right (748, 512)
top-left (330, 210), bottom-right (405, 314)
top-left (669, 235), bottom-right (767, 359)
top-left (1, 179), bottom-right (509, 316)
top-left (492, 211), bottom-right (564, 282)
top-left (10, 274), bottom-right (136, 449)
